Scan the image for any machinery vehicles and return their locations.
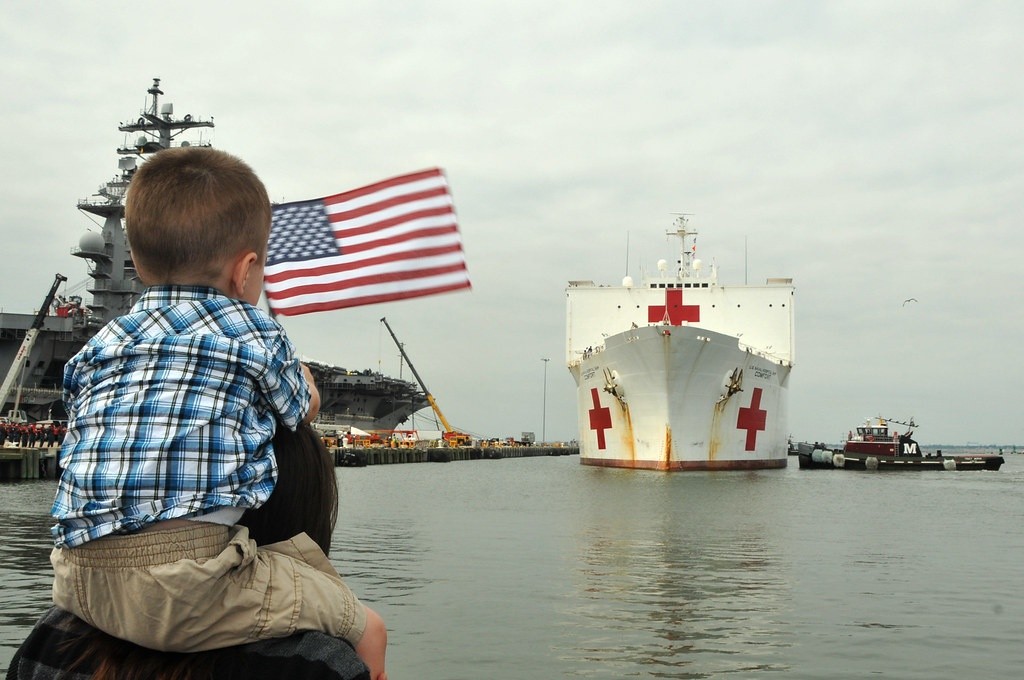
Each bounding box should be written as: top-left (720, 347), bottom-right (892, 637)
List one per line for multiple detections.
top-left (379, 316), bottom-right (473, 448)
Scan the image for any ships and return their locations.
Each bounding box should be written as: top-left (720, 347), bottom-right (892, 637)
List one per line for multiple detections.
top-left (0, 71), bottom-right (433, 440)
top-left (564, 210), bottom-right (795, 474)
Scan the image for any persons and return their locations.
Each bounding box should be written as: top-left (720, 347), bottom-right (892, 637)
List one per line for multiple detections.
top-left (7, 423), bottom-right (386, 680)
top-left (49, 141), bottom-right (387, 680)
top-left (1, 418), bottom-right (63, 448)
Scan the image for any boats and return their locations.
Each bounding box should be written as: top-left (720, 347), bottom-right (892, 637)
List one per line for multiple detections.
top-left (798, 415), bottom-right (1006, 471)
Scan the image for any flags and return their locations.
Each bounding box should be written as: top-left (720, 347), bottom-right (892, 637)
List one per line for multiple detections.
top-left (260, 165), bottom-right (472, 318)
